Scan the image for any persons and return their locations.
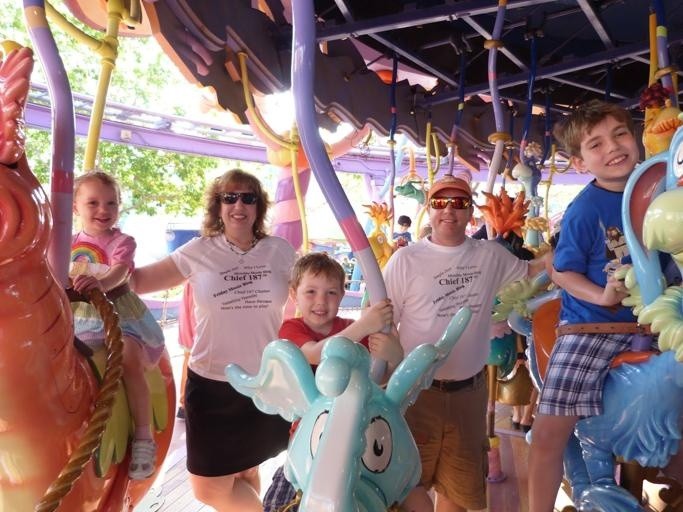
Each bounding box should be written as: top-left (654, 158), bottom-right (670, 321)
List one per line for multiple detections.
top-left (62, 168), bottom-right (166, 479)
top-left (506, 125), bottom-right (682, 512)
top-left (511, 335), bottom-right (535, 434)
top-left (123, 168), bottom-right (301, 510)
top-left (172, 281), bottom-right (199, 419)
top-left (379, 174), bottom-right (563, 511)
top-left (392, 213), bottom-right (410, 242)
top-left (259, 253), bottom-right (404, 510)
top-left (523, 94), bottom-right (646, 510)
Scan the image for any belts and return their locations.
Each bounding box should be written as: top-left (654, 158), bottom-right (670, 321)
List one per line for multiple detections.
top-left (554, 321), bottom-right (651, 338)
top-left (433, 365), bottom-right (486, 391)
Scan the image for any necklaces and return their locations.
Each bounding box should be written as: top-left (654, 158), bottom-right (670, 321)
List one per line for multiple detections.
top-left (225, 238), bottom-right (259, 255)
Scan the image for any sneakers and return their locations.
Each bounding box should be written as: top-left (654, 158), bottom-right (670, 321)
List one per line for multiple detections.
top-left (128, 440), bottom-right (157, 480)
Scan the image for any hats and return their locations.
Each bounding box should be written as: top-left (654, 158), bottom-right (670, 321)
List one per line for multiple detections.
top-left (426, 174), bottom-right (472, 201)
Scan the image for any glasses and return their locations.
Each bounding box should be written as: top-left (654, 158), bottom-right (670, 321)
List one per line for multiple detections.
top-left (428, 195), bottom-right (472, 210)
top-left (217, 191), bottom-right (259, 205)
